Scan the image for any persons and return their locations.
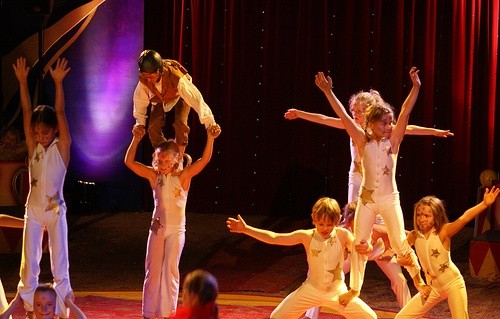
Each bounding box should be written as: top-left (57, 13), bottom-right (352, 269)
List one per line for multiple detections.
top-left (303, 201), bottom-right (411, 319)
top-left (124, 123), bottom-right (221, 319)
top-left (132, 50), bottom-right (221, 171)
top-left (393, 186), bottom-right (500, 319)
top-left (183, 270), bottom-right (219, 319)
top-left (0, 285), bottom-right (87, 319)
top-left (13, 58), bottom-right (72, 319)
top-left (226, 196), bottom-right (377, 319)
top-left (0, 128), bottom-right (19, 160)
top-left (0, 213), bottom-right (47, 319)
top-left (284, 90), bottom-right (454, 262)
top-left (317, 66), bottom-right (431, 306)
